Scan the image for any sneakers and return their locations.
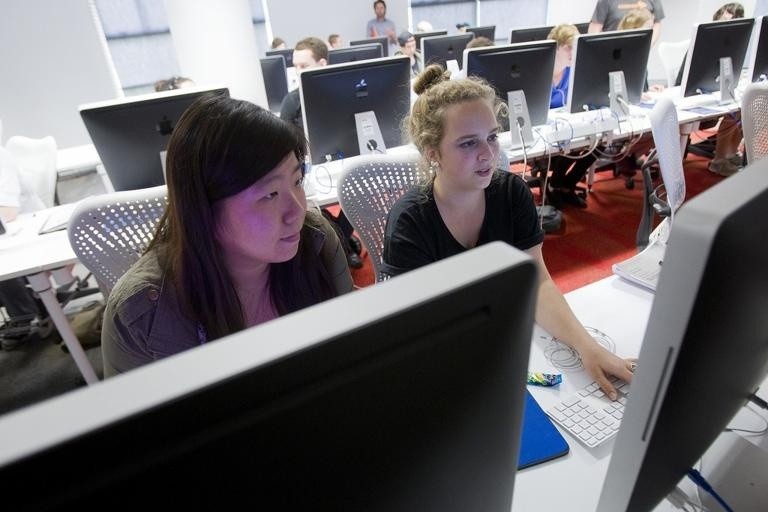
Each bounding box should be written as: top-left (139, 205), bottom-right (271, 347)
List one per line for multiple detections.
top-left (544, 179), bottom-right (587, 211)
top-left (709, 151), bottom-right (748, 177)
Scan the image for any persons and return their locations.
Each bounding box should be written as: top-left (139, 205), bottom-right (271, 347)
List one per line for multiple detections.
top-left (102, 92), bottom-right (356, 382)
top-left (586, 0), bottom-right (664, 93)
top-left (152, 77), bottom-right (197, 92)
top-left (278, 37), bottom-right (330, 157)
top-left (630, 362), bottom-right (638, 373)
top-left (547, 23), bottom-right (604, 208)
top-left (415, 21), bottom-right (433, 33)
top-left (618, 10), bottom-right (654, 176)
top-left (269, 37), bottom-right (286, 50)
top-left (466, 37), bottom-right (494, 51)
top-left (380, 64), bottom-right (640, 402)
top-left (394, 32), bottom-right (423, 79)
top-left (366, 0), bottom-right (395, 58)
top-left (456, 22), bottom-right (469, 32)
top-left (674, 3), bottom-right (744, 176)
top-left (327, 34), bottom-right (342, 52)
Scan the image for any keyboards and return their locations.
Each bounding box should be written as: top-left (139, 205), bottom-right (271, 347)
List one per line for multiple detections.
top-left (543, 359), bottom-right (637, 451)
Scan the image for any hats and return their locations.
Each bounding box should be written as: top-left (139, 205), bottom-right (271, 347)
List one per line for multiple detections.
top-left (397, 32), bottom-right (417, 45)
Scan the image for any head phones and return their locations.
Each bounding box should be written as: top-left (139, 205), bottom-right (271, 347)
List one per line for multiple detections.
top-left (168, 77), bottom-right (178, 90)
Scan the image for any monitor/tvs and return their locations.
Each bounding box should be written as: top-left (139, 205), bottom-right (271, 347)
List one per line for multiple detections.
top-left (465, 25), bottom-right (497, 42)
top-left (679, 17), bottom-right (754, 105)
top-left (510, 26), bottom-right (556, 42)
top-left (298, 54), bottom-right (413, 166)
top-left (351, 37), bottom-right (390, 57)
top-left (78, 86), bottom-right (232, 192)
top-left (750, 16), bottom-right (767, 82)
top-left (413, 31), bottom-right (448, 49)
top-left (595, 156), bottom-right (767, 511)
top-left (565, 27), bottom-right (655, 124)
top-left (266, 49), bottom-right (294, 67)
top-left (259, 55), bottom-right (288, 113)
top-left (328, 42), bottom-right (384, 67)
top-left (576, 23), bottom-right (590, 33)
top-left (0, 237), bottom-right (544, 511)
top-left (420, 33), bottom-right (467, 75)
top-left (462, 39), bottom-right (559, 153)
